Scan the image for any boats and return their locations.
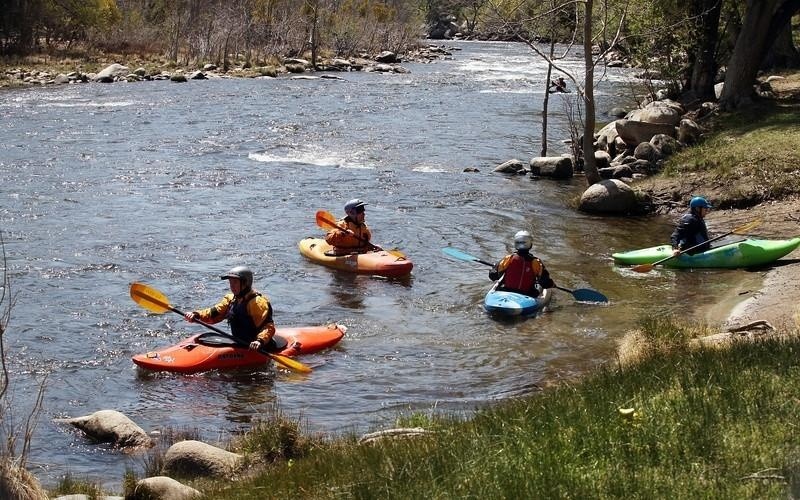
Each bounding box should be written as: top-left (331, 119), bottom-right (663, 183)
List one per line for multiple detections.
top-left (297, 234), bottom-right (415, 278)
top-left (611, 234), bottom-right (800, 270)
top-left (550, 84), bottom-right (570, 94)
top-left (480, 274), bottom-right (552, 323)
top-left (128, 322), bottom-right (348, 374)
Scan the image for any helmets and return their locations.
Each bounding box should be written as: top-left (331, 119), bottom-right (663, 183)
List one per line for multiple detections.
top-left (345, 200), bottom-right (368, 223)
top-left (221, 267), bottom-right (252, 295)
top-left (515, 231), bottom-right (533, 251)
top-left (690, 198), bottom-right (713, 209)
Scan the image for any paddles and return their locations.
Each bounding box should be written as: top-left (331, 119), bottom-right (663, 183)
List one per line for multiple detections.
top-left (316, 210), bottom-right (403, 258)
top-left (631, 221), bottom-right (762, 273)
top-left (443, 246), bottom-right (609, 304)
top-left (130, 282), bottom-right (311, 373)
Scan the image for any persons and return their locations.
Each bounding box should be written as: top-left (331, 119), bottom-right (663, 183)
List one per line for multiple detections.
top-left (489, 230), bottom-right (553, 297)
top-left (184, 266), bottom-right (275, 351)
top-left (553, 77), bottom-right (566, 88)
top-left (670, 197), bottom-right (710, 254)
top-left (325, 200), bottom-right (371, 255)
